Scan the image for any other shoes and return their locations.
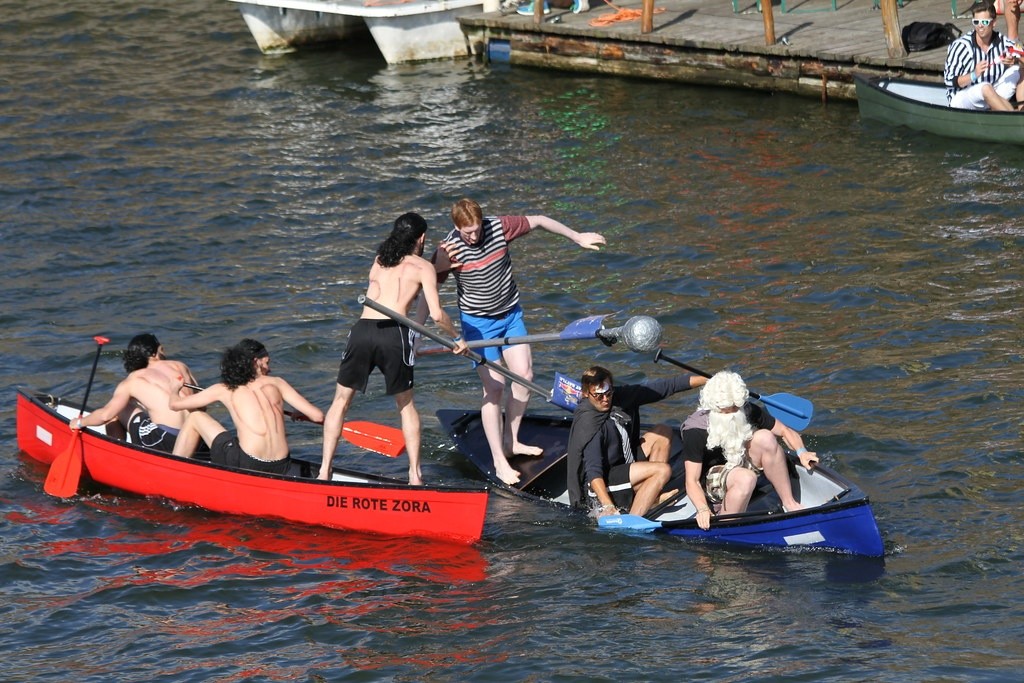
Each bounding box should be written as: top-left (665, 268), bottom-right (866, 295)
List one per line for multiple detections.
top-left (569, 0), bottom-right (591, 13)
top-left (516, 0), bottom-right (551, 16)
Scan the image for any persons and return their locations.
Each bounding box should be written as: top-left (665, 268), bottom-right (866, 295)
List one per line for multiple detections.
top-left (67, 333), bottom-right (201, 456)
top-left (679, 371), bottom-right (820, 530)
top-left (1000, 0), bottom-right (1024, 50)
top-left (943, 2), bottom-right (1024, 111)
top-left (517, 0), bottom-right (590, 15)
top-left (430, 199), bottom-right (608, 486)
top-left (567, 366), bottom-right (713, 521)
top-left (317, 212), bottom-right (471, 486)
top-left (168, 338), bottom-right (325, 477)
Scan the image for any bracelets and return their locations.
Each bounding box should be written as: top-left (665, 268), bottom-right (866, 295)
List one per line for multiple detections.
top-left (795, 447), bottom-right (808, 455)
top-left (971, 70), bottom-right (976, 84)
top-left (76, 418), bottom-right (82, 429)
top-left (452, 334), bottom-right (462, 341)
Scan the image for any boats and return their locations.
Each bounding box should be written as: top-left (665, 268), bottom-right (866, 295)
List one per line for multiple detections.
top-left (433, 410), bottom-right (885, 562)
top-left (852, 71), bottom-right (1024, 143)
top-left (15, 385), bottom-right (489, 543)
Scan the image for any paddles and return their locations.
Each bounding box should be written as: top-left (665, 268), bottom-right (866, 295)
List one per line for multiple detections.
top-left (653, 348), bottom-right (814, 433)
top-left (43, 335), bottom-right (112, 498)
top-left (177, 372), bottom-right (407, 459)
top-left (596, 507), bottom-right (781, 533)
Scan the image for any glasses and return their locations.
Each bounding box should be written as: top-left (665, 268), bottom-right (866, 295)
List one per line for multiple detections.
top-left (588, 386), bottom-right (614, 401)
top-left (971, 18), bottom-right (993, 27)
